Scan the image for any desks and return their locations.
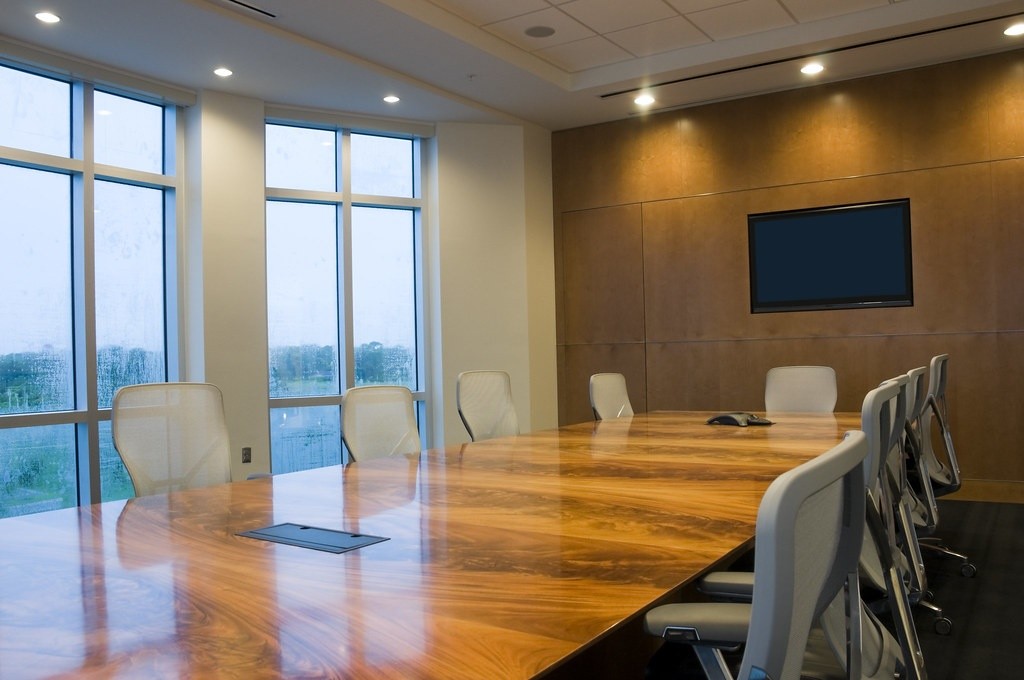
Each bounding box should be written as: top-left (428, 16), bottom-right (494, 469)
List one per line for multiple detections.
top-left (0, 410), bottom-right (866, 678)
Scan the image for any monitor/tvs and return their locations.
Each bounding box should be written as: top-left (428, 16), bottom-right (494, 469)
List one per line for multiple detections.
top-left (747, 197), bottom-right (914, 315)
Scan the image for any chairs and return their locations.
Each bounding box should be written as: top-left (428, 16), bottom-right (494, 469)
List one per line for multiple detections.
top-left (110, 383), bottom-right (276, 498)
top-left (696, 383), bottom-right (954, 636)
top-left (870, 353), bottom-right (977, 579)
top-left (765, 365), bottom-right (837, 412)
top-left (340, 386), bottom-right (422, 463)
top-left (456, 370), bottom-right (521, 443)
top-left (589, 372), bottom-right (634, 421)
top-left (643, 429), bottom-right (930, 680)
